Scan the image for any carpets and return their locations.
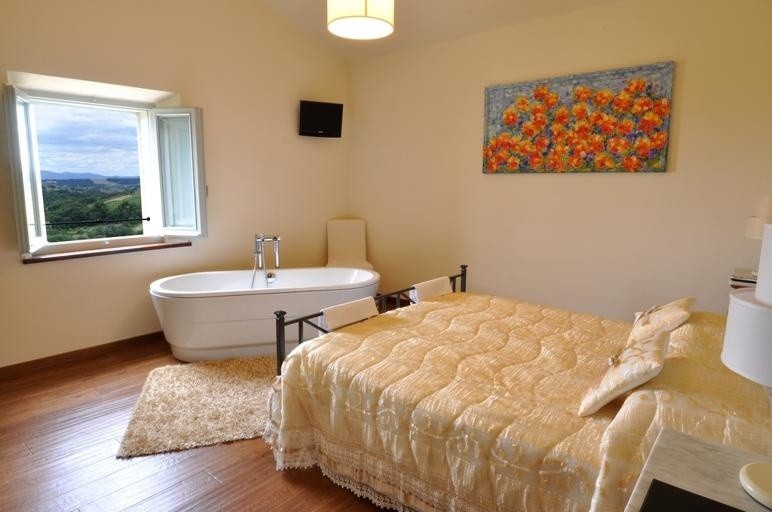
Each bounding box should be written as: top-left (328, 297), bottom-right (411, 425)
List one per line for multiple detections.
top-left (109, 357), bottom-right (294, 465)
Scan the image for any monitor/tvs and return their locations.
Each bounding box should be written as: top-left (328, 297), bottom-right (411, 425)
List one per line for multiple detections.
top-left (299, 100), bottom-right (343, 137)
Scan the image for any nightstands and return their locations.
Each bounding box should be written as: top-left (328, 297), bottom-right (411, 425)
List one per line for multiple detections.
top-left (623, 426), bottom-right (772, 512)
top-left (728, 267), bottom-right (757, 290)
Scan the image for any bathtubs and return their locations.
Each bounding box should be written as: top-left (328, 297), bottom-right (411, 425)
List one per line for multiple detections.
top-left (149, 267), bottom-right (381, 363)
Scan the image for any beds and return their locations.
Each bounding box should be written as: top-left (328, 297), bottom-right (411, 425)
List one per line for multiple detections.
top-left (272, 258), bottom-right (772, 512)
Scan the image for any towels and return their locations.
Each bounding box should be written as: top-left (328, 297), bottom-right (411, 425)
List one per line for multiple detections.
top-left (408, 272), bottom-right (453, 304)
top-left (318, 295), bottom-right (379, 340)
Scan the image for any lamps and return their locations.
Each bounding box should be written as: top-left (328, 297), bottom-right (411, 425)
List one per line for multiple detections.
top-left (744, 217), bottom-right (772, 276)
top-left (722, 223), bottom-right (772, 510)
top-left (325, 1), bottom-right (395, 41)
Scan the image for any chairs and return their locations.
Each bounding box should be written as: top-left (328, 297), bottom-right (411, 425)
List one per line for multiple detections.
top-left (324, 216), bottom-right (374, 271)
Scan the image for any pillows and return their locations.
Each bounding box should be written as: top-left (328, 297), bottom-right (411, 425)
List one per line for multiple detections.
top-left (626, 296), bottom-right (697, 343)
top-left (579, 328), bottom-right (670, 421)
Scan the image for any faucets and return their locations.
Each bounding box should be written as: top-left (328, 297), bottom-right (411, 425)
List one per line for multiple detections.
top-left (255, 234), bottom-right (280, 267)
